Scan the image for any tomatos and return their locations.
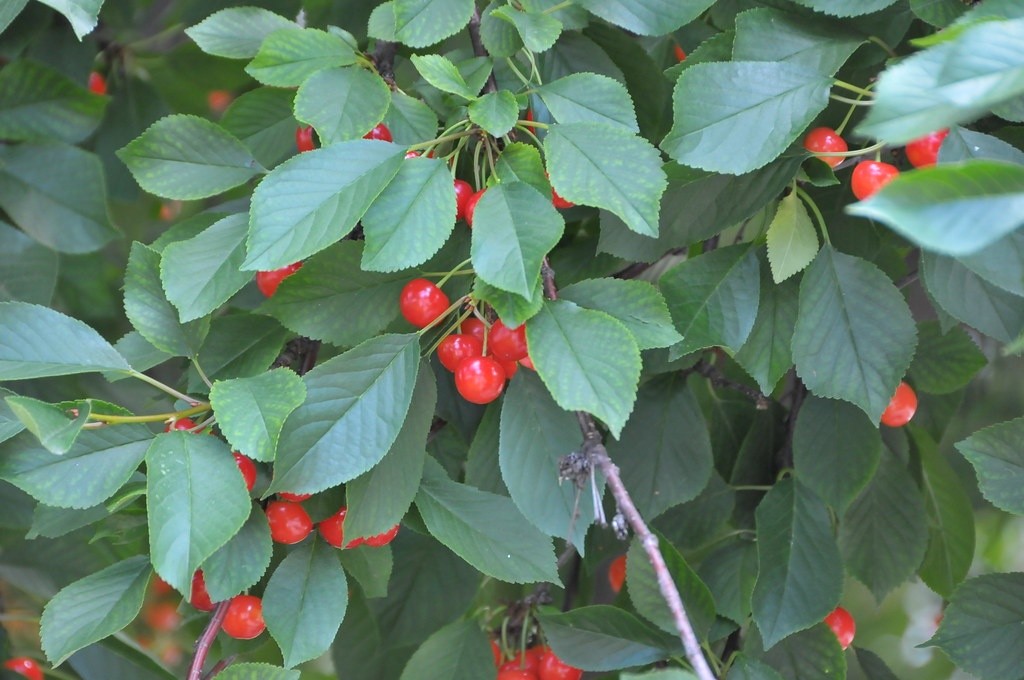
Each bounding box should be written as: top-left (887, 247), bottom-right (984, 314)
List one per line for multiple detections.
top-left (0, 37), bottom-right (948, 679)
